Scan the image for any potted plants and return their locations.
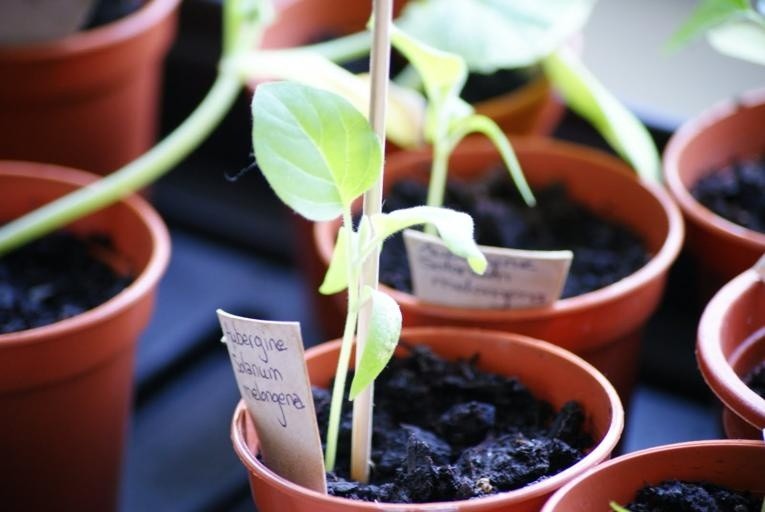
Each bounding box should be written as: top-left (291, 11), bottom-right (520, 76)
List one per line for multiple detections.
top-left (1, 0), bottom-right (765, 511)
